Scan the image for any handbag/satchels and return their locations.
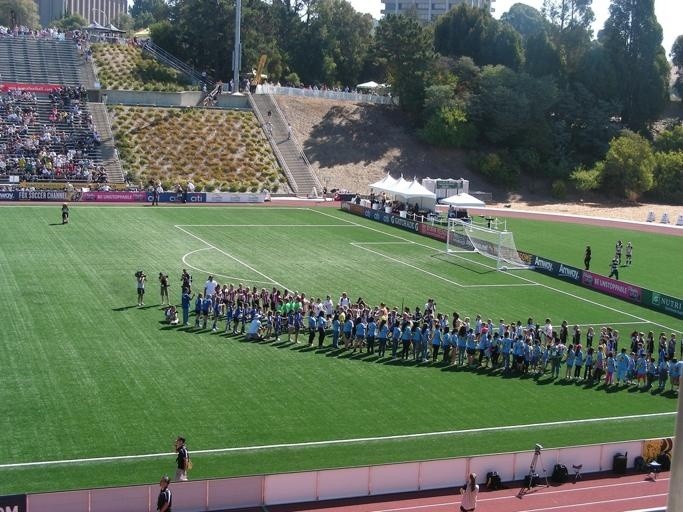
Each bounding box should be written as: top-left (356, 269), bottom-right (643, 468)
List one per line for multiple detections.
top-left (183, 459), bottom-right (193, 471)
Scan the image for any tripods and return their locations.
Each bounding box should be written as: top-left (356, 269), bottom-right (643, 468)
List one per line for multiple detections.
top-left (528, 451), bottom-right (549, 490)
top-left (575, 473), bottom-right (583, 480)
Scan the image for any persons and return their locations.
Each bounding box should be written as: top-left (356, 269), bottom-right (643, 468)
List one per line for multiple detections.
top-left (287, 123), bottom-right (292, 141)
top-left (135, 270), bottom-right (145, 307)
top-left (173, 435), bottom-right (190, 482)
top-left (202, 74), bottom-right (373, 109)
top-left (0, 16), bottom-right (194, 208)
top-left (267, 121), bottom-right (273, 136)
top-left (59, 199), bottom-right (68, 226)
top-left (608, 254), bottom-right (619, 280)
top-left (459, 472), bottom-right (479, 512)
top-left (624, 241), bottom-right (633, 265)
top-left (265, 111), bottom-right (272, 125)
top-left (318, 186), bottom-right (430, 223)
top-left (583, 245), bottom-right (592, 270)
top-left (162, 306), bottom-right (178, 326)
top-left (180, 265), bottom-right (683, 399)
top-left (157, 271), bottom-right (171, 306)
top-left (156, 474), bottom-right (173, 512)
top-left (615, 240), bottom-right (623, 264)
